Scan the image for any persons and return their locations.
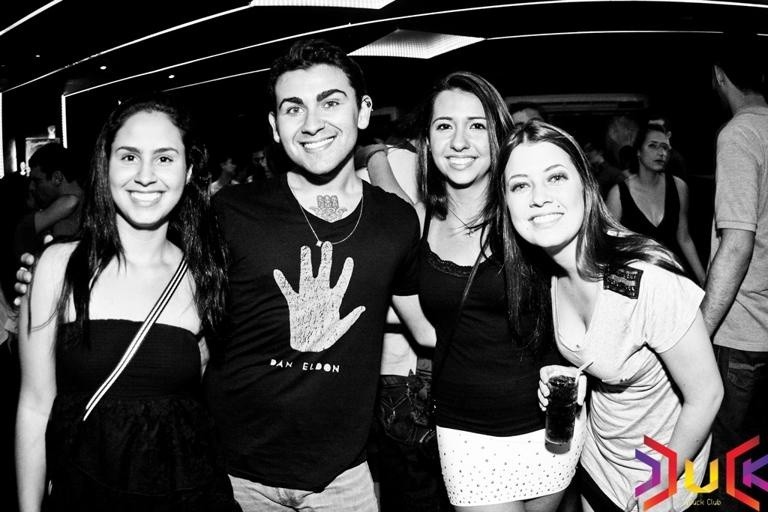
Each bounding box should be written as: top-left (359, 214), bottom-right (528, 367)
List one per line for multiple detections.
top-left (1, 100), bottom-right (768, 511)
top-left (698, 15), bottom-right (766, 508)
top-left (405, 67), bottom-right (595, 511)
top-left (14, 32), bottom-right (440, 512)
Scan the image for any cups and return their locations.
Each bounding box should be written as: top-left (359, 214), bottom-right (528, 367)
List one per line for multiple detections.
top-left (543, 374), bottom-right (579, 456)
